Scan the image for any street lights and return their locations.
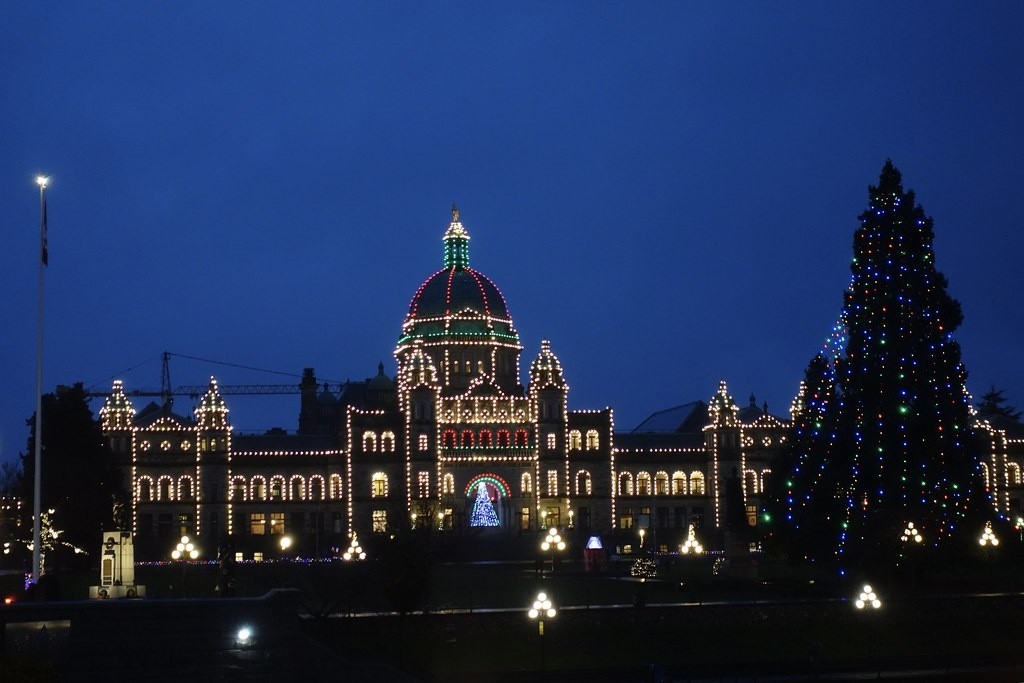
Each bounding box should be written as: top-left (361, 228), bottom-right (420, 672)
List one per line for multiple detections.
top-left (539, 528), bottom-right (567, 579)
top-left (528, 591), bottom-right (557, 670)
top-left (854, 583), bottom-right (882, 660)
top-left (170, 536), bottom-right (202, 600)
top-left (680, 530), bottom-right (704, 582)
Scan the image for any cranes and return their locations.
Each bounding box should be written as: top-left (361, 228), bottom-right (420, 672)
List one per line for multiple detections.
top-left (82, 349), bottom-right (346, 406)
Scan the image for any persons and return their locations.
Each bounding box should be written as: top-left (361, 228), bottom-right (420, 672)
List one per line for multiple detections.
top-left (532, 544), bottom-right (549, 578)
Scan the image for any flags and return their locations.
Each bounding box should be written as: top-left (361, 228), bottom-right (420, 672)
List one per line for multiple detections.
top-left (43, 201), bottom-right (47, 265)
